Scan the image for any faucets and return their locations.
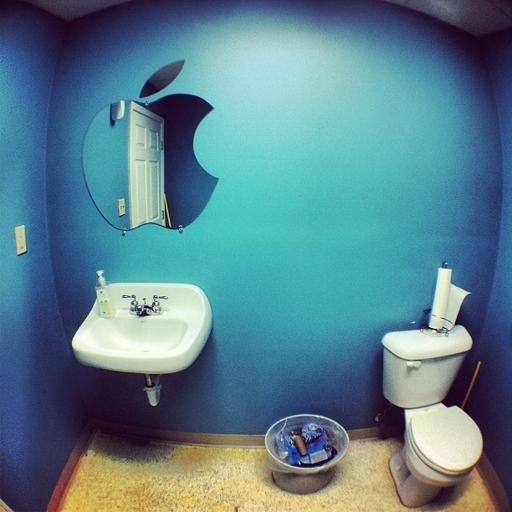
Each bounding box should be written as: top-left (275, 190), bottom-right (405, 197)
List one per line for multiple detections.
top-left (136, 304), bottom-right (153, 317)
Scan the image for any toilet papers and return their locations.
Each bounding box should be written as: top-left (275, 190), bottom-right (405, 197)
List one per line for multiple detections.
top-left (428, 267), bottom-right (471, 331)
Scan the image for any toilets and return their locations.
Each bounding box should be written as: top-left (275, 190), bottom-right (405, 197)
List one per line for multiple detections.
top-left (379, 323), bottom-right (484, 509)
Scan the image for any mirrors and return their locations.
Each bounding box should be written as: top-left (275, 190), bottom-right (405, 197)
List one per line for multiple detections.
top-left (80, 60), bottom-right (219, 235)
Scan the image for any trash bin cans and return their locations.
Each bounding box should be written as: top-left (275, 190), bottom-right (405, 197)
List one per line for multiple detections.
top-left (265, 414), bottom-right (350, 496)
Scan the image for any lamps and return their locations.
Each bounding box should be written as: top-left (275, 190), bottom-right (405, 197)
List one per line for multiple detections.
top-left (110, 99), bottom-right (125, 122)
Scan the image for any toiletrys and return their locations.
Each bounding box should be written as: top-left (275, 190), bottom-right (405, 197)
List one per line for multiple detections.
top-left (93, 269), bottom-right (118, 319)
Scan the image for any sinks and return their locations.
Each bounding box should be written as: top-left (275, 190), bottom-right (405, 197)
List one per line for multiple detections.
top-left (69, 283), bottom-right (213, 377)
